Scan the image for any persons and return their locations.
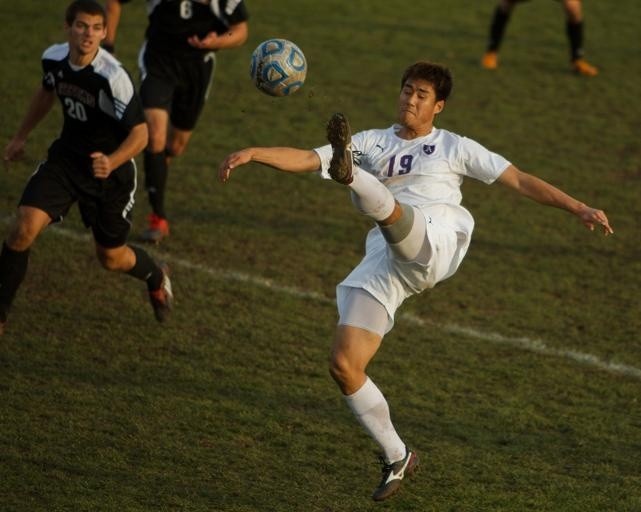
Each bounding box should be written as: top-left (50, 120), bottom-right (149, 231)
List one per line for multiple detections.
top-left (482, 0), bottom-right (600, 80)
top-left (221, 61), bottom-right (613, 501)
top-left (104, 1), bottom-right (249, 244)
top-left (0, 0), bottom-right (173, 322)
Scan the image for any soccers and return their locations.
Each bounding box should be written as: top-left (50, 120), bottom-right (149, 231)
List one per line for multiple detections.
top-left (250, 39), bottom-right (306, 97)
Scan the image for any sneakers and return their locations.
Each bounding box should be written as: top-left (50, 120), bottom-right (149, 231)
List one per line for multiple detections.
top-left (144, 210), bottom-right (171, 249)
top-left (325, 110), bottom-right (355, 187)
top-left (371, 444), bottom-right (421, 503)
top-left (480, 49), bottom-right (499, 72)
top-left (570, 56), bottom-right (599, 79)
top-left (147, 265), bottom-right (176, 323)
